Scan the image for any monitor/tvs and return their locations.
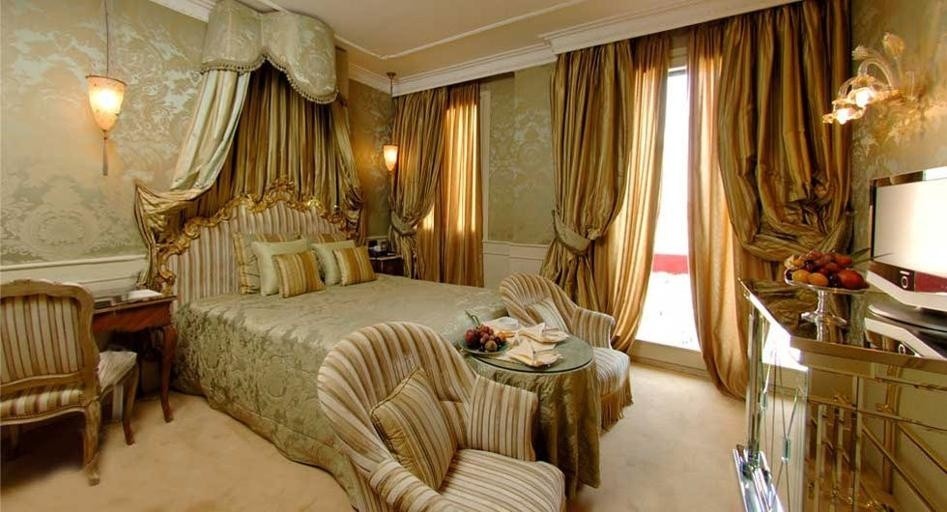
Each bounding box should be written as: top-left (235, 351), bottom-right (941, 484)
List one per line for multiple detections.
top-left (865, 164), bottom-right (946, 332)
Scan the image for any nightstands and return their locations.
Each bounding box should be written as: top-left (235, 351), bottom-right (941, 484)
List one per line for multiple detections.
top-left (370, 255), bottom-right (404, 276)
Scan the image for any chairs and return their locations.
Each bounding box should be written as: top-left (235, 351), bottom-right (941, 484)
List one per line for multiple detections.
top-left (500, 272), bottom-right (633, 429)
top-left (0, 279), bottom-right (138, 487)
top-left (318, 322), bottom-right (565, 512)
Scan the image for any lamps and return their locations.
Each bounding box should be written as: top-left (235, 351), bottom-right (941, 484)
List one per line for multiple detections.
top-left (83, 0), bottom-right (126, 176)
top-left (824, 31), bottom-right (929, 124)
top-left (384, 72), bottom-right (400, 172)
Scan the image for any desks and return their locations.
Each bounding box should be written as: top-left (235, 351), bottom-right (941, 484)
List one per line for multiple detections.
top-left (92, 285), bottom-right (174, 422)
top-left (732, 277), bottom-right (947, 512)
top-left (458, 327), bottom-right (600, 501)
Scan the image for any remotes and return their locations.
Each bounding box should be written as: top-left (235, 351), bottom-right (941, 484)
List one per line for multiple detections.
top-left (752, 278), bottom-right (799, 294)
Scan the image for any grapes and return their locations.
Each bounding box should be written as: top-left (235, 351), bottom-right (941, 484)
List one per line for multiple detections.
top-left (474, 324), bottom-right (500, 352)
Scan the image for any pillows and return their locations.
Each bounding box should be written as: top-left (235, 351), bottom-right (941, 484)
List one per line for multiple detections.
top-left (251, 239), bottom-right (308, 297)
top-left (231, 231), bottom-right (304, 293)
top-left (301, 231), bottom-right (345, 282)
top-left (313, 240), bottom-right (356, 286)
top-left (332, 244), bottom-right (377, 286)
top-left (369, 368), bottom-right (456, 490)
top-left (527, 295), bottom-right (569, 333)
top-left (272, 248), bottom-right (326, 298)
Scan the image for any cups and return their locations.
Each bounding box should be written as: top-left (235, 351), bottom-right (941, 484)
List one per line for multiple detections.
top-left (497, 316), bottom-right (519, 329)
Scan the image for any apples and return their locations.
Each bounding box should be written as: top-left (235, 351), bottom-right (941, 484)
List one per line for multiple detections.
top-left (465, 329), bottom-right (478, 348)
top-left (837, 270), bottom-right (865, 290)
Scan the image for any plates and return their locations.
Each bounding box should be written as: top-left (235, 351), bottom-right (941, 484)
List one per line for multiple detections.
top-left (458, 336), bottom-right (510, 354)
top-left (547, 337), bottom-right (566, 343)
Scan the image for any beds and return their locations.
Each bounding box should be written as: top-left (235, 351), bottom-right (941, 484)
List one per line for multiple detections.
top-left (153, 174), bottom-right (504, 512)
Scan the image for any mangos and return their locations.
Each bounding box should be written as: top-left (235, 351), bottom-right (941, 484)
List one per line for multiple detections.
top-left (808, 273), bottom-right (829, 286)
top-left (792, 269), bottom-right (811, 283)
